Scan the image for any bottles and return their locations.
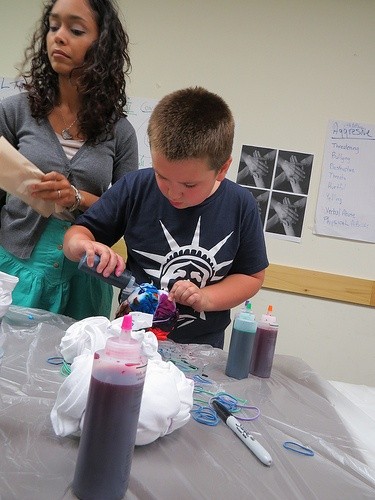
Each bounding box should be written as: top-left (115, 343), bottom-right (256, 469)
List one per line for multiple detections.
top-left (78, 252), bottom-right (139, 289)
top-left (249, 305), bottom-right (279, 378)
top-left (226, 303), bottom-right (258, 380)
top-left (72, 315), bottom-right (148, 500)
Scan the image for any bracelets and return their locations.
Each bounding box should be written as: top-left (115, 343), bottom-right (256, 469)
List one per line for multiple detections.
top-left (63, 185), bottom-right (81, 212)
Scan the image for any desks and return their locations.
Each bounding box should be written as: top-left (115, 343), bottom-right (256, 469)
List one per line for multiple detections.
top-left (0, 304), bottom-right (375, 500)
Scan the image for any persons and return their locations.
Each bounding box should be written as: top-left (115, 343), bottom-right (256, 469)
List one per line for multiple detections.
top-left (235, 150), bottom-right (315, 239)
top-left (62, 87), bottom-right (269, 350)
top-left (0, 0), bottom-right (139, 319)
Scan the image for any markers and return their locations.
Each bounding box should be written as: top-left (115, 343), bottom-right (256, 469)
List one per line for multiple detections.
top-left (212, 399), bottom-right (274, 466)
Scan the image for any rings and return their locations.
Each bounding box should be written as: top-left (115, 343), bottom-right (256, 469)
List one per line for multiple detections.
top-left (57, 189), bottom-right (61, 200)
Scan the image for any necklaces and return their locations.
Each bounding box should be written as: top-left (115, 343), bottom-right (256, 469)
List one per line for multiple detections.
top-left (54, 107), bottom-right (80, 140)
